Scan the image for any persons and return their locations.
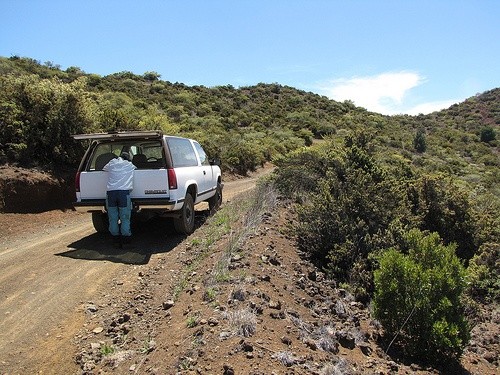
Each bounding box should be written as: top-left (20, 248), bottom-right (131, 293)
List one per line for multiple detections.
top-left (103, 151), bottom-right (137, 241)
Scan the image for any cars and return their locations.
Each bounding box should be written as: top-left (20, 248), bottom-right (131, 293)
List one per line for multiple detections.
top-left (69, 127), bottom-right (225, 237)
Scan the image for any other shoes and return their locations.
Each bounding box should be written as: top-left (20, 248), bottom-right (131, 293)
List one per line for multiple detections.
top-left (110, 235), bottom-right (135, 243)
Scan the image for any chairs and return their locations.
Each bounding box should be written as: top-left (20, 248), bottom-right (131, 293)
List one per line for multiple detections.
top-left (133, 153), bottom-right (149, 168)
top-left (95, 152), bottom-right (120, 170)
